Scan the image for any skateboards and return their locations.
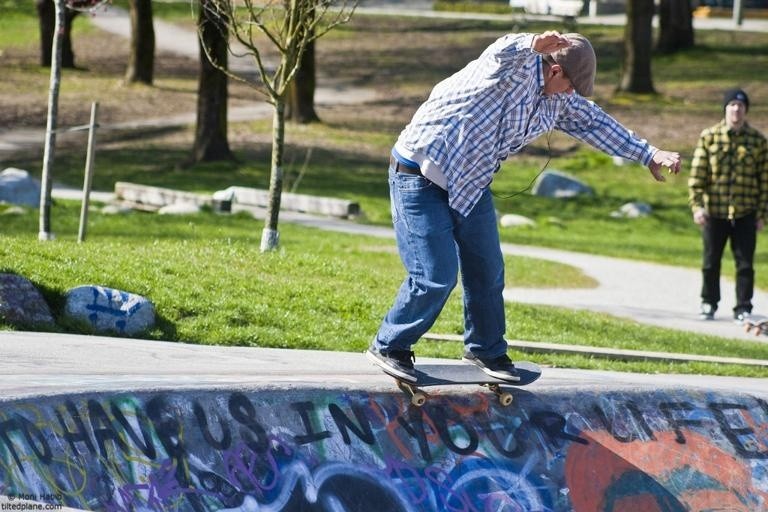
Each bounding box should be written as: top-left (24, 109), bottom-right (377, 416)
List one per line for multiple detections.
top-left (380, 360), bottom-right (541, 407)
top-left (741, 310), bottom-right (767, 338)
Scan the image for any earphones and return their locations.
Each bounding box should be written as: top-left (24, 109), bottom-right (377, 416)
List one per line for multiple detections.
top-left (552, 71), bottom-right (558, 77)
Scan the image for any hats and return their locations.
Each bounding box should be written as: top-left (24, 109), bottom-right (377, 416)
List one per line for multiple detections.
top-left (549, 33), bottom-right (596, 96)
top-left (724, 89), bottom-right (749, 114)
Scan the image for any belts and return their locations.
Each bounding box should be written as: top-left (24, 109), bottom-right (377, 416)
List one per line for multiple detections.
top-left (389, 153), bottom-right (422, 174)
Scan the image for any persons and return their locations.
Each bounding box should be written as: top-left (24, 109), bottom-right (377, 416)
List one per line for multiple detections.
top-left (367, 30), bottom-right (681, 383)
top-left (688, 88), bottom-right (767, 325)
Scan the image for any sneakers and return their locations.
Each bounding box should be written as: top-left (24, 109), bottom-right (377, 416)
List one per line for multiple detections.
top-left (365, 344), bottom-right (419, 385)
top-left (701, 302), bottom-right (715, 316)
top-left (737, 312), bottom-right (749, 322)
top-left (462, 347), bottom-right (521, 382)
